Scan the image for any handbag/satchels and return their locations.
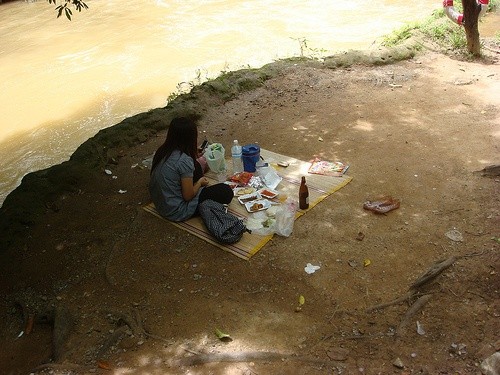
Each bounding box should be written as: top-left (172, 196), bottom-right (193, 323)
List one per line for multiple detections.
top-left (199, 199), bottom-right (252, 245)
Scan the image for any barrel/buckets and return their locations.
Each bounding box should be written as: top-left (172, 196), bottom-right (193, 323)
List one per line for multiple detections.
top-left (242, 144), bottom-right (264, 173)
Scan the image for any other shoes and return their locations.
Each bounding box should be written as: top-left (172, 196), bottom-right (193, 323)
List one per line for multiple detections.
top-left (363, 195), bottom-right (400, 214)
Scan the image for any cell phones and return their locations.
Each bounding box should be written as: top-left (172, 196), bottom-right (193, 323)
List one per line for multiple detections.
top-left (200, 141), bottom-right (208, 153)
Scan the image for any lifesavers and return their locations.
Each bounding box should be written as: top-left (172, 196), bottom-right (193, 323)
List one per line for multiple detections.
top-left (443, 0), bottom-right (488, 26)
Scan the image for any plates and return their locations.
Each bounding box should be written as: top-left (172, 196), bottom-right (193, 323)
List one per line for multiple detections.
top-left (224, 181), bottom-right (237, 190)
top-left (238, 191), bottom-right (262, 204)
top-left (234, 188), bottom-right (255, 196)
top-left (259, 188), bottom-right (278, 198)
top-left (245, 199), bottom-right (271, 212)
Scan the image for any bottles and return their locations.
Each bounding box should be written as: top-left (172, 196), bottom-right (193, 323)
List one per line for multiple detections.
top-left (299, 176), bottom-right (309, 210)
top-left (231, 140), bottom-right (243, 173)
top-left (218, 153), bottom-right (226, 183)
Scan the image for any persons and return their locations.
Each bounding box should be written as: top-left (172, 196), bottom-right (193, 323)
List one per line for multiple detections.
top-left (149, 115), bottom-right (233, 222)
